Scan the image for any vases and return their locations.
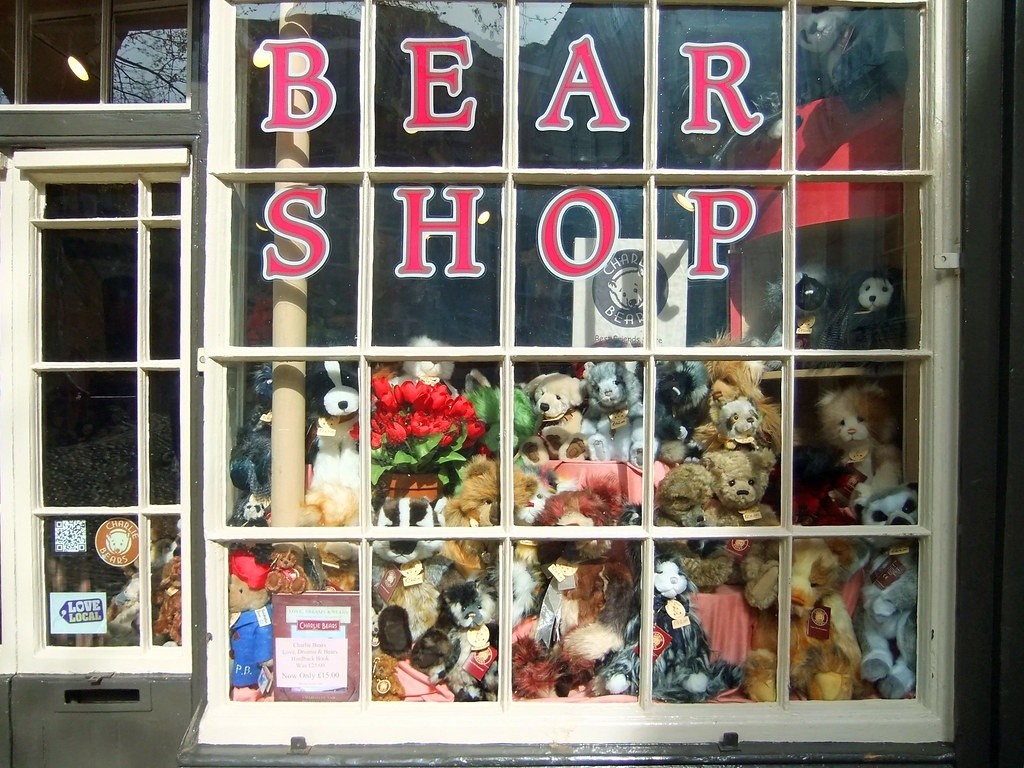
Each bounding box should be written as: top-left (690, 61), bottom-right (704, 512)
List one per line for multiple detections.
top-left (379, 472), bottom-right (445, 505)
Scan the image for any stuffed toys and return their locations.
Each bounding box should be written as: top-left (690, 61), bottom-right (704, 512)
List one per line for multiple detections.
top-left (110, 266), bottom-right (918, 702)
top-left (673, 80), bottom-right (782, 169)
top-left (796, 4), bottom-right (909, 112)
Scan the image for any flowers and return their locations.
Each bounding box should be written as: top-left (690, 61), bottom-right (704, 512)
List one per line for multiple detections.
top-left (349, 376), bottom-right (493, 488)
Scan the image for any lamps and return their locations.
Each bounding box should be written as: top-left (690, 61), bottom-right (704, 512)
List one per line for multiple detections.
top-left (68, 33), bottom-right (122, 81)
